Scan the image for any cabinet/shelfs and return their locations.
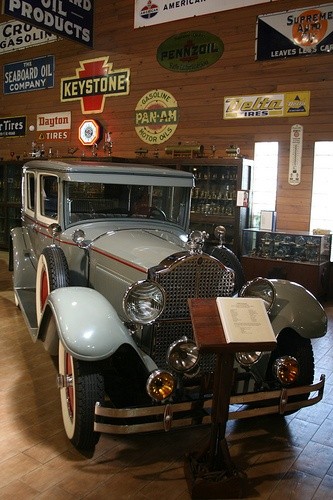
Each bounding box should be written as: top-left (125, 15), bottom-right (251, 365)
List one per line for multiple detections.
top-left (0, 157), bottom-right (332, 302)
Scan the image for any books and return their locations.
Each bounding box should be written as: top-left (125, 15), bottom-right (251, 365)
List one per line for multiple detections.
top-left (216, 297), bottom-right (277, 343)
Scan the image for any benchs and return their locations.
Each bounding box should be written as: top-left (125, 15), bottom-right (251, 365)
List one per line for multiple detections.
top-left (71, 198), bottom-right (127, 214)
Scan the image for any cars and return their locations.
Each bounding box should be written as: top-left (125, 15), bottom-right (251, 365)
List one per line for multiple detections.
top-left (8, 158), bottom-right (329, 447)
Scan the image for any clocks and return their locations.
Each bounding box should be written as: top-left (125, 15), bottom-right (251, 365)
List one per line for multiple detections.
top-left (78, 119), bottom-right (103, 146)
